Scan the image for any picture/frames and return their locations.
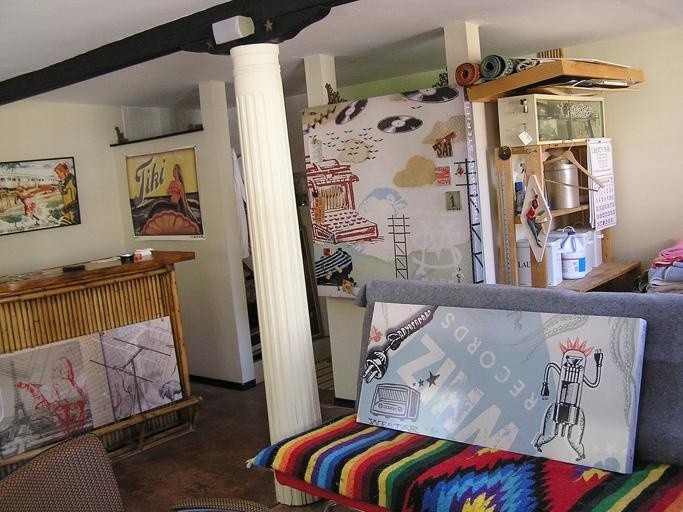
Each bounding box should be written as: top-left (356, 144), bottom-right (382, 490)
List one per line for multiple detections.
top-left (0, 156), bottom-right (82, 236)
top-left (125, 146), bottom-right (205, 242)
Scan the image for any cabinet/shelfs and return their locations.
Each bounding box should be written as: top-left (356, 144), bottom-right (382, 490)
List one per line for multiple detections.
top-left (500, 143), bottom-right (641, 291)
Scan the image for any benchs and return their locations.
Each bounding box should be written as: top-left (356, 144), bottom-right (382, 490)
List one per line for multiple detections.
top-left (273, 278), bottom-right (683, 511)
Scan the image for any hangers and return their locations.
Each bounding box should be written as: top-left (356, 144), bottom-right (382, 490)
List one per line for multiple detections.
top-left (543, 139), bottom-right (603, 192)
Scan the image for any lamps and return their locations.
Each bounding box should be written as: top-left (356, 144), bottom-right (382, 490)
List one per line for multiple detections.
top-left (212, 14), bottom-right (256, 44)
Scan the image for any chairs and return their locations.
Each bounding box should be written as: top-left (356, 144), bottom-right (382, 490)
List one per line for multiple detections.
top-left (1, 432), bottom-right (271, 512)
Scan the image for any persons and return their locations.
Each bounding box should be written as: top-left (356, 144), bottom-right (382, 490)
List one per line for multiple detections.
top-left (50, 163), bottom-right (80, 226)
top-left (15, 185), bottom-right (40, 221)
top-left (139, 164), bottom-right (198, 226)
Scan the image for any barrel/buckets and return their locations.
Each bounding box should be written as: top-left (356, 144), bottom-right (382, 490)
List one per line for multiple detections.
top-left (561, 231), bottom-right (587, 280)
top-left (545, 163), bottom-right (580, 210)
